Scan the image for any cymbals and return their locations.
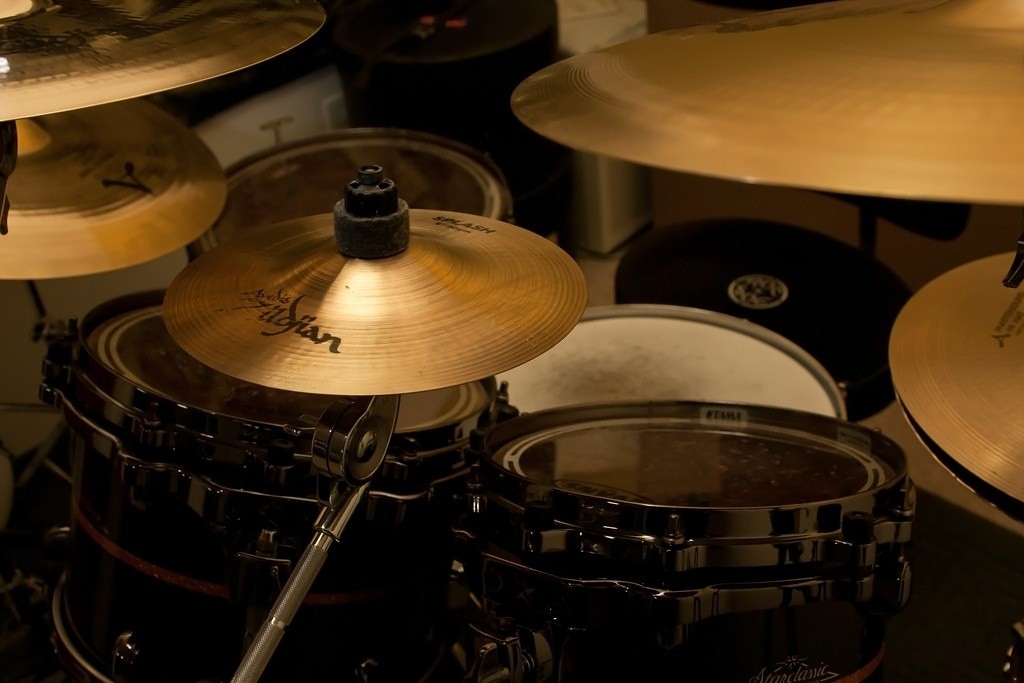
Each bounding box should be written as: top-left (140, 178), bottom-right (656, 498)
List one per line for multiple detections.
top-left (0, 0), bottom-right (325, 124)
top-left (512, 0), bottom-right (1024, 205)
top-left (0, 100), bottom-right (225, 281)
top-left (164, 206), bottom-right (588, 397)
top-left (888, 251), bottom-right (1024, 505)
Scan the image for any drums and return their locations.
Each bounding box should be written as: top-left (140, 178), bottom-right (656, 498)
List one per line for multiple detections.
top-left (472, 399), bottom-right (916, 683)
top-left (489, 298), bottom-right (847, 426)
top-left (37, 290), bottom-right (485, 683)
top-left (183, 125), bottom-right (509, 260)
